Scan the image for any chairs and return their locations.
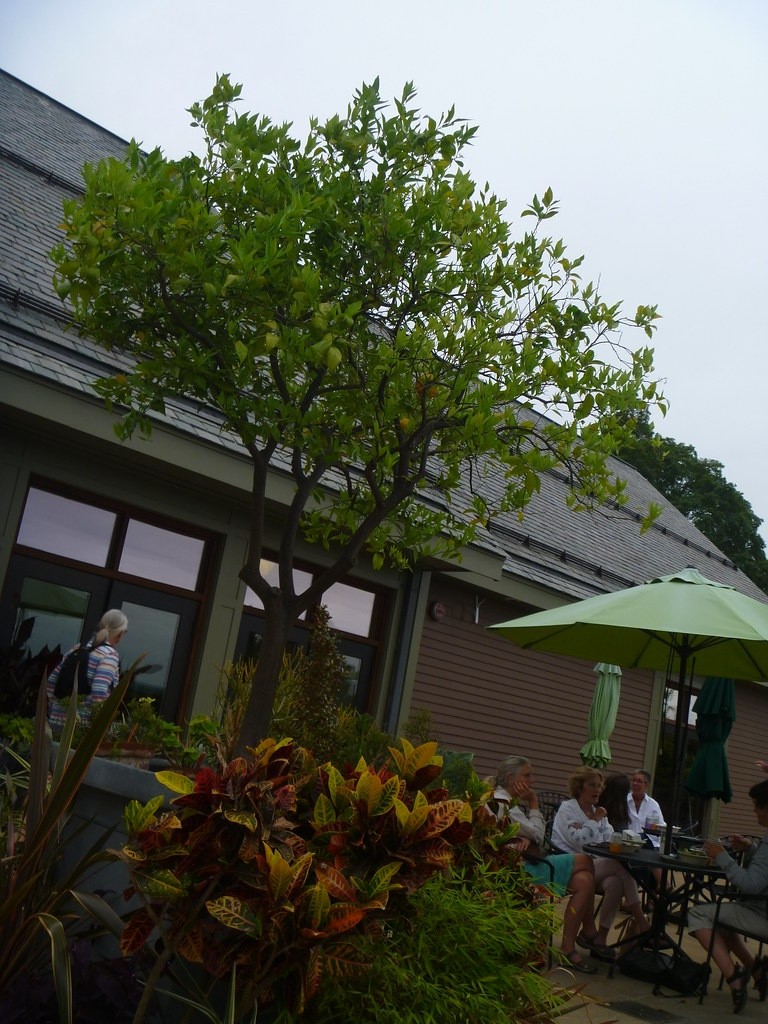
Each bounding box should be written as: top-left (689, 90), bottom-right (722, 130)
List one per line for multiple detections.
top-left (521, 790), bottom-right (768, 1006)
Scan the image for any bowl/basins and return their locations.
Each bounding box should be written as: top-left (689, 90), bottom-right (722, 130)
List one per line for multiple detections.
top-left (622, 840), bottom-right (647, 854)
top-left (677, 851), bottom-right (711, 866)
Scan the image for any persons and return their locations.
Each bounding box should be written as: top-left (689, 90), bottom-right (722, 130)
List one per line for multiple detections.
top-left (484, 755), bottom-right (616, 973)
top-left (40, 609), bottom-right (129, 784)
top-left (688, 780), bottom-right (768, 1014)
top-left (551, 767), bottom-right (679, 958)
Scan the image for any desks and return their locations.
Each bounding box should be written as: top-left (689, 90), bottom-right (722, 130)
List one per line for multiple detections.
top-left (582, 843), bottom-right (726, 991)
top-left (642, 827), bottom-right (731, 927)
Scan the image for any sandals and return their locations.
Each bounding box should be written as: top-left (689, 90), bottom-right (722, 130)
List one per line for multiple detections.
top-left (577, 929), bottom-right (617, 958)
top-left (751, 955), bottom-right (768, 1002)
top-left (725, 961), bottom-right (751, 1014)
top-left (557, 950), bottom-right (598, 973)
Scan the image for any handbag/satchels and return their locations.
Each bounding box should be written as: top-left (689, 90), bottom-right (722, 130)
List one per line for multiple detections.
top-left (519, 837), bottom-right (548, 865)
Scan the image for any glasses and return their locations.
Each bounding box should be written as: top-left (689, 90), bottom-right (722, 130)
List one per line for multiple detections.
top-left (631, 777), bottom-right (646, 784)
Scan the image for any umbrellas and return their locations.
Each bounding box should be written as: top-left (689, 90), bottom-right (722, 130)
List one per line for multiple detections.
top-left (579, 662), bottom-right (622, 770)
top-left (484, 566), bottom-right (768, 964)
top-left (683, 677), bottom-right (736, 837)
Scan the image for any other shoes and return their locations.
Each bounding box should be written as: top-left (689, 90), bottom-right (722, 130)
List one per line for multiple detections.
top-left (641, 927), bottom-right (670, 948)
top-left (590, 948), bottom-right (620, 962)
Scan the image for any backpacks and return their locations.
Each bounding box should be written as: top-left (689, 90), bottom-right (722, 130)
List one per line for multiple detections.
top-left (53, 640), bottom-right (116, 700)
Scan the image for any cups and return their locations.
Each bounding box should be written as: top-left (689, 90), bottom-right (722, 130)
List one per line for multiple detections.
top-left (609, 832), bottom-right (622, 853)
